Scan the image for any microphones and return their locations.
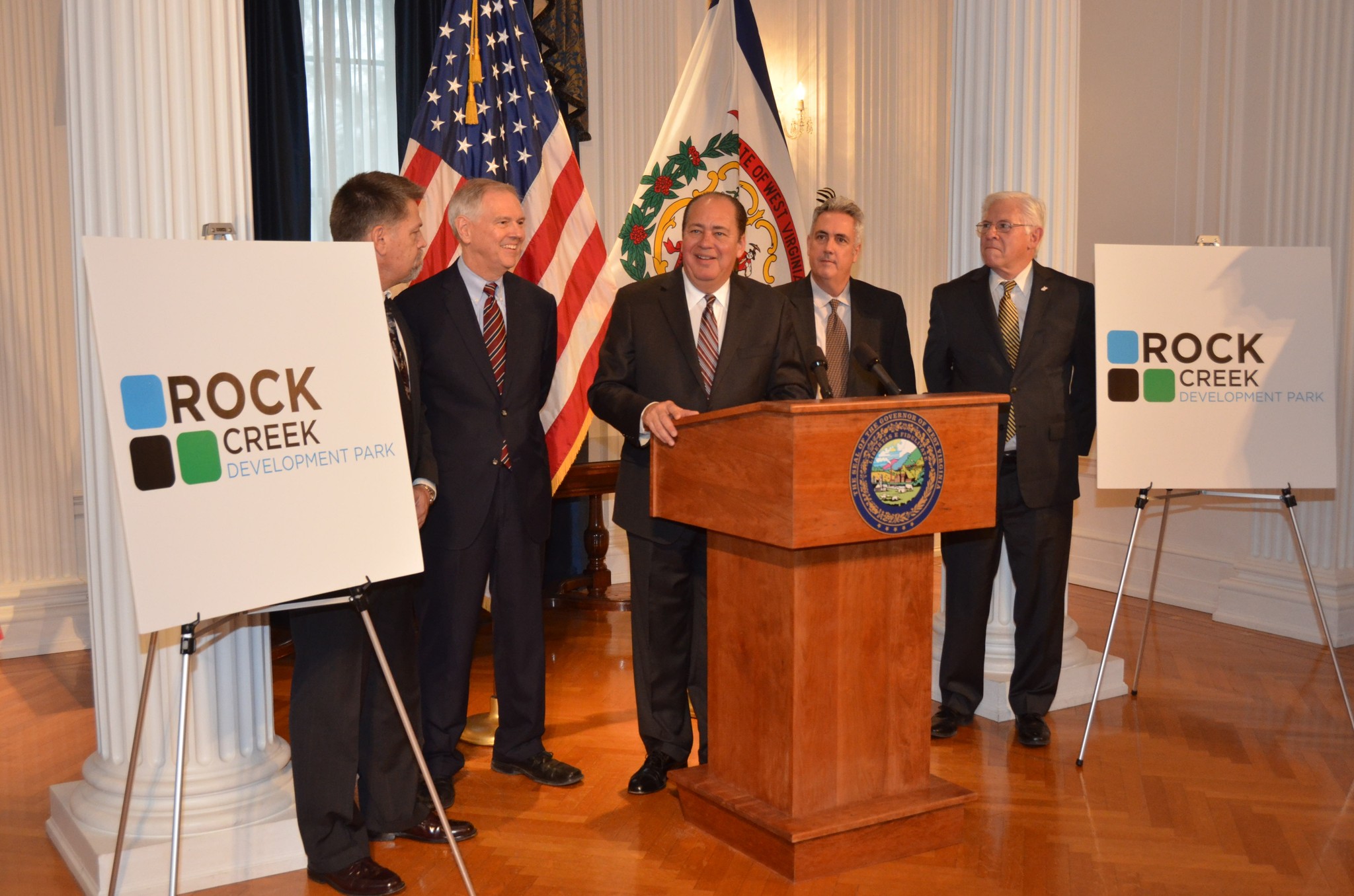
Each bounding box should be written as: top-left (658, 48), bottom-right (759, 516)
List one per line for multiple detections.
top-left (806, 346), bottom-right (833, 398)
top-left (852, 342), bottom-right (900, 394)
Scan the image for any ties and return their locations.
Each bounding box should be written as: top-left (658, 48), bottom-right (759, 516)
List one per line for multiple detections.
top-left (697, 295), bottom-right (719, 397)
top-left (999, 281), bottom-right (1020, 442)
top-left (384, 294), bottom-right (411, 402)
top-left (483, 283), bottom-right (512, 471)
top-left (826, 300), bottom-right (849, 398)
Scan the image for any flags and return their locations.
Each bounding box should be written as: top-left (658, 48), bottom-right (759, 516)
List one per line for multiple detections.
top-left (396, 0), bottom-right (626, 614)
top-left (608, 2), bottom-right (816, 290)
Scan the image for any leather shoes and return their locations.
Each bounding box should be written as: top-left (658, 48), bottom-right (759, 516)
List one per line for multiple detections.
top-left (628, 753), bottom-right (687, 794)
top-left (931, 705), bottom-right (975, 738)
top-left (1015, 715), bottom-right (1050, 745)
top-left (414, 775), bottom-right (456, 810)
top-left (491, 749), bottom-right (585, 785)
top-left (307, 855), bottom-right (405, 896)
top-left (367, 810), bottom-right (478, 841)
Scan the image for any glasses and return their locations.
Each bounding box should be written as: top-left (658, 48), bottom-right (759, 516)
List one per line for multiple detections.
top-left (976, 220), bottom-right (1036, 234)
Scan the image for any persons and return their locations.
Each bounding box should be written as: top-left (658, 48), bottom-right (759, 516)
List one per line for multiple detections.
top-left (921, 189), bottom-right (1097, 748)
top-left (287, 171), bottom-right (478, 896)
top-left (770, 195), bottom-right (919, 399)
top-left (392, 177), bottom-right (585, 810)
top-left (587, 190), bottom-right (814, 796)
